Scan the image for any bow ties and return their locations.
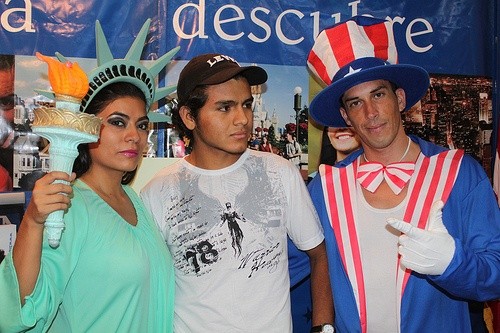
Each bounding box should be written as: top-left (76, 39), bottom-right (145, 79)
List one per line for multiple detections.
top-left (357, 161), bottom-right (415, 195)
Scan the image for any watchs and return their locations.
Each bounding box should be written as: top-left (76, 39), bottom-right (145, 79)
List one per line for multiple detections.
top-left (311, 323), bottom-right (336, 333)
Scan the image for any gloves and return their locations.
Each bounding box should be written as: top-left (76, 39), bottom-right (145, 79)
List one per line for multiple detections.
top-left (387, 200), bottom-right (456, 275)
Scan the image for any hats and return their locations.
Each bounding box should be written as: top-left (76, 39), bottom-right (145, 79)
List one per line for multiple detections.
top-left (33, 17), bottom-right (180, 122)
top-left (307, 16), bottom-right (430, 128)
top-left (177, 54), bottom-right (268, 106)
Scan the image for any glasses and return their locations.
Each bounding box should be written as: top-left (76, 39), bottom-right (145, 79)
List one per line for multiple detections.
top-left (0, 94), bottom-right (14, 110)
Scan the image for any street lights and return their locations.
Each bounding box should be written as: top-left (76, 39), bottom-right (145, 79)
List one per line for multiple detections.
top-left (293, 85), bottom-right (304, 145)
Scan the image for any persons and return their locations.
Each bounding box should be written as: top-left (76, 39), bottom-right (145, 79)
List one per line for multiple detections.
top-left (182, 132), bottom-right (302, 172)
top-left (317, 125), bottom-right (362, 170)
top-left (0, 18), bottom-right (175, 333)
top-left (139, 53), bottom-right (336, 333)
top-left (287, 15), bottom-right (500, 333)
